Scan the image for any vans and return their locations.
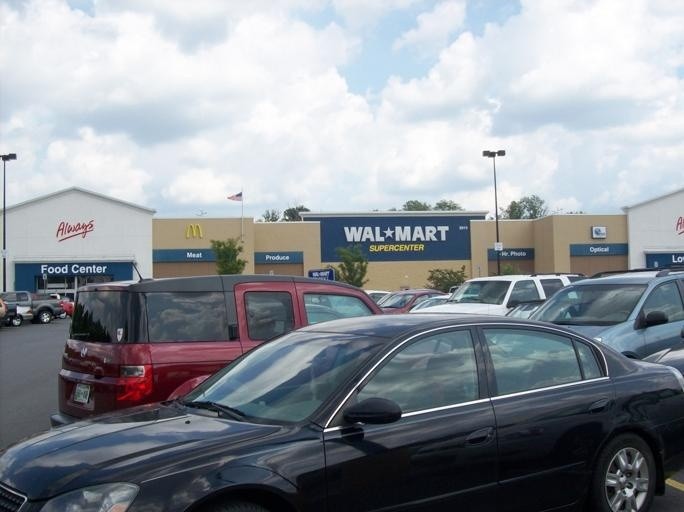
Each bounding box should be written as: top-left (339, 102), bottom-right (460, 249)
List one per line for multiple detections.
top-left (46, 259), bottom-right (391, 429)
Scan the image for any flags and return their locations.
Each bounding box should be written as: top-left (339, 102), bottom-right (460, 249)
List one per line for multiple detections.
top-left (227, 192), bottom-right (241, 201)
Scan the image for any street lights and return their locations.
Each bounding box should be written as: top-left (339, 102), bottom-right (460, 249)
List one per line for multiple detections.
top-left (482, 149), bottom-right (507, 277)
top-left (0, 153), bottom-right (17, 292)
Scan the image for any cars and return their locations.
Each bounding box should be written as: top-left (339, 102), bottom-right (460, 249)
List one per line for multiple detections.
top-left (0, 311), bottom-right (683, 512)
top-left (0, 291), bottom-right (76, 327)
